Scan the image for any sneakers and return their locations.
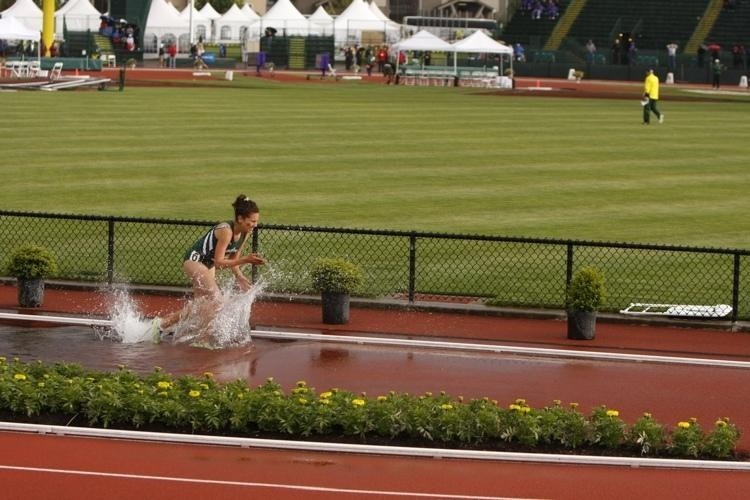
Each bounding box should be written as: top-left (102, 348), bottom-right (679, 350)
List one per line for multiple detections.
top-left (659, 115), bottom-right (664, 123)
top-left (194, 342), bottom-right (223, 349)
top-left (152, 319), bottom-right (163, 344)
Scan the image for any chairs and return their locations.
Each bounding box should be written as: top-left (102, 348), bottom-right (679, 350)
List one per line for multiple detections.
top-left (0, 60), bottom-right (63, 81)
top-left (405, 70), bottom-right (498, 89)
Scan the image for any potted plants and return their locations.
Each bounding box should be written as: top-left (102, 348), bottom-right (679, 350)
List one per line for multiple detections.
top-left (566, 266), bottom-right (608, 340)
top-left (350, 65), bottom-right (360, 73)
top-left (505, 68), bottom-right (512, 78)
top-left (5, 243), bottom-right (62, 307)
top-left (310, 256), bottom-right (361, 324)
top-left (573, 71), bottom-right (584, 81)
top-left (127, 58), bottom-right (137, 69)
top-left (265, 62), bottom-right (274, 72)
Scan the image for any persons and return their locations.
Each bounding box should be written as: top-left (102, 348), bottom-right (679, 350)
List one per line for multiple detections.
top-left (572, 62), bottom-right (585, 81)
top-left (637, 63), bottom-right (665, 125)
top-left (711, 58), bottom-right (726, 89)
top-left (159, 43), bottom-right (166, 67)
top-left (26, 43), bottom-right (37, 57)
top-left (342, 41), bottom-right (409, 76)
top-left (586, 40), bottom-right (597, 64)
top-left (610, 39), bottom-right (623, 63)
top-left (320, 50), bottom-right (329, 81)
top-left (196, 35), bottom-right (211, 69)
top-left (98, 16), bottom-right (138, 54)
top-left (151, 193), bottom-right (269, 353)
top-left (15, 39), bottom-right (24, 58)
top-left (731, 38), bottom-right (746, 65)
top-left (59, 42), bottom-right (68, 56)
top-left (264, 52), bottom-right (277, 74)
top-left (618, 33), bottom-right (628, 64)
top-left (519, 0), bottom-right (561, 21)
top-left (515, 43), bottom-right (527, 63)
top-left (665, 42), bottom-right (678, 71)
top-left (627, 38), bottom-right (638, 68)
top-left (49, 43), bottom-right (58, 58)
top-left (167, 38), bottom-right (177, 69)
top-left (707, 41), bottom-right (720, 60)
top-left (220, 44), bottom-right (226, 58)
top-left (423, 51), bottom-right (430, 65)
top-left (696, 44), bottom-right (706, 68)
top-left (241, 42), bottom-right (249, 70)
